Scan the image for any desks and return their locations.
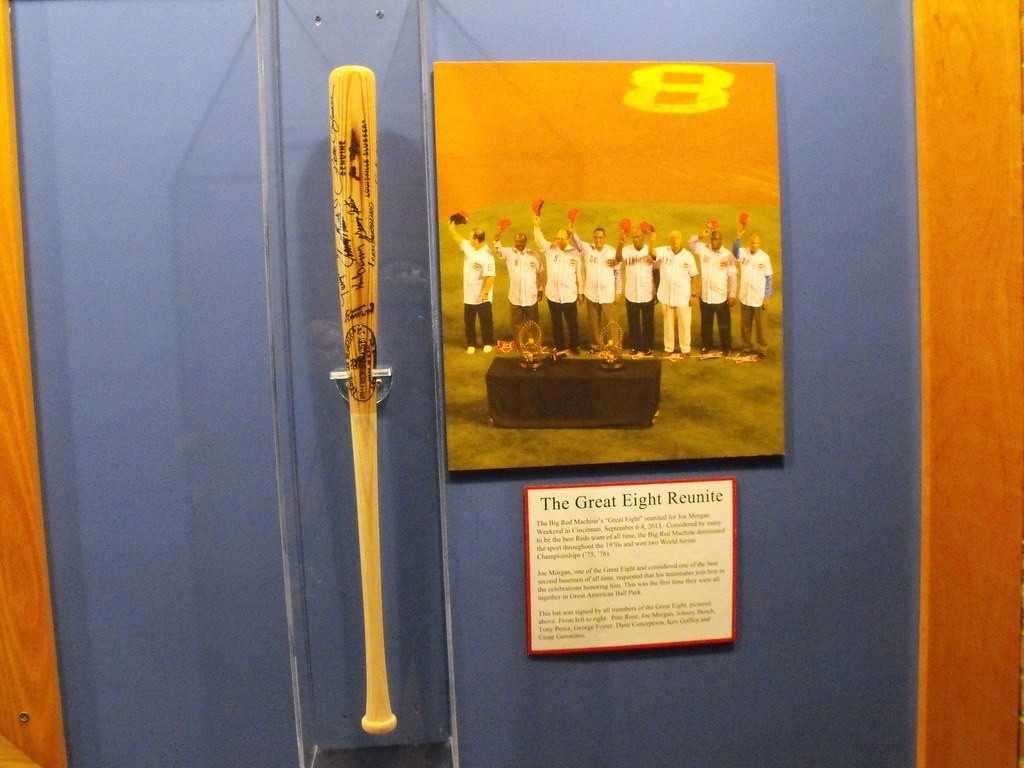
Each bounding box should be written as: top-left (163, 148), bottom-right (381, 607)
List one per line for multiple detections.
top-left (485, 356), bottom-right (661, 429)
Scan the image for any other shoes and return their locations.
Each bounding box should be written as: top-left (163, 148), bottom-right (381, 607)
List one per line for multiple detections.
top-left (554, 344), bottom-right (566, 352)
top-left (645, 348), bottom-right (652, 356)
top-left (723, 349), bottom-right (729, 357)
top-left (757, 352), bottom-right (766, 359)
top-left (662, 352), bottom-right (672, 357)
top-left (568, 346), bottom-right (580, 355)
top-left (483, 344), bottom-right (493, 353)
top-left (700, 347), bottom-right (712, 354)
top-left (681, 352), bottom-right (690, 359)
top-left (740, 351), bottom-right (751, 357)
top-left (467, 346), bottom-right (475, 355)
top-left (630, 347), bottom-right (640, 355)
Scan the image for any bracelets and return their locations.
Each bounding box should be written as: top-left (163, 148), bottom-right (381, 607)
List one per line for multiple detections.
top-left (691, 294), bottom-right (698, 297)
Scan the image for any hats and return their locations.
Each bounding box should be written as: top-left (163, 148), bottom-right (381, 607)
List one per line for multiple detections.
top-left (705, 218), bottom-right (721, 231)
top-left (640, 221), bottom-right (655, 234)
top-left (449, 212), bottom-right (467, 225)
top-left (740, 213), bottom-right (748, 230)
top-left (498, 218), bottom-right (512, 235)
top-left (620, 218), bottom-right (631, 237)
top-left (532, 199), bottom-right (544, 216)
top-left (567, 208), bottom-right (578, 228)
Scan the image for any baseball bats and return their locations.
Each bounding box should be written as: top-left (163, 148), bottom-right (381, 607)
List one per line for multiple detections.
top-left (323, 62), bottom-right (397, 737)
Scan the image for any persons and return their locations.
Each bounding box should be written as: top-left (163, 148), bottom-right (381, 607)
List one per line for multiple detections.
top-left (688, 231), bottom-right (738, 358)
top-left (732, 231), bottom-right (773, 357)
top-left (649, 230), bottom-right (699, 359)
top-left (534, 215), bottom-right (584, 353)
top-left (569, 225), bottom-right (622, 350)
top-left (493, 232), bottom-right (544, 341)
top-left (448, 220), bottom-right (496, 355)
top-left (618, 229), bottom-right (660, 354)
